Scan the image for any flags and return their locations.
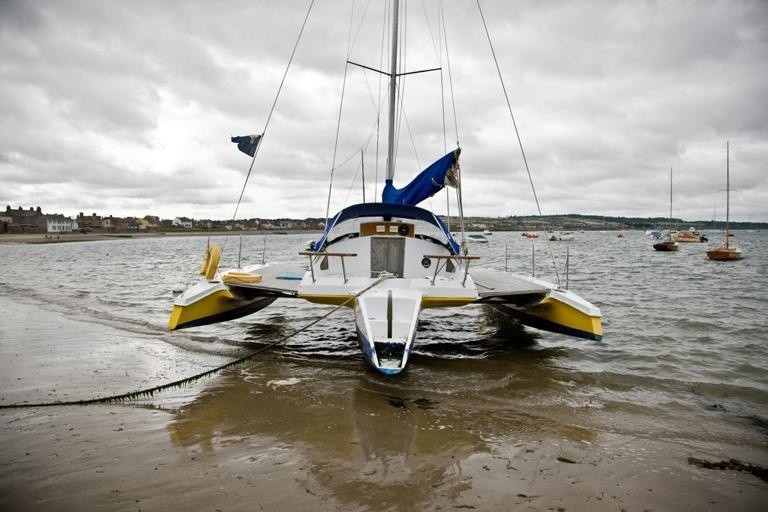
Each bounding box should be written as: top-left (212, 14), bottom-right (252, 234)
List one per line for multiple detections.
top-left (232, 134), bottom-right (261, 157)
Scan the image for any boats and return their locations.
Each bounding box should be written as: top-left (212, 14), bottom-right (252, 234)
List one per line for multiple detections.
top-left (462, 234), bottom-right (490, 245)
top-left (548, 230), bottom-right (578, 242)
top-left (672, 230), bottom-right (710, 244)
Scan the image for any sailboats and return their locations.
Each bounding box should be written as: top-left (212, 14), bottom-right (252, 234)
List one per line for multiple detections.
top-left (170, 0), bottom-right (608, 381)
top-left (705, 138), bottom-right (748, 261)
top-left (651, 165), bottom-right (681, 252)
